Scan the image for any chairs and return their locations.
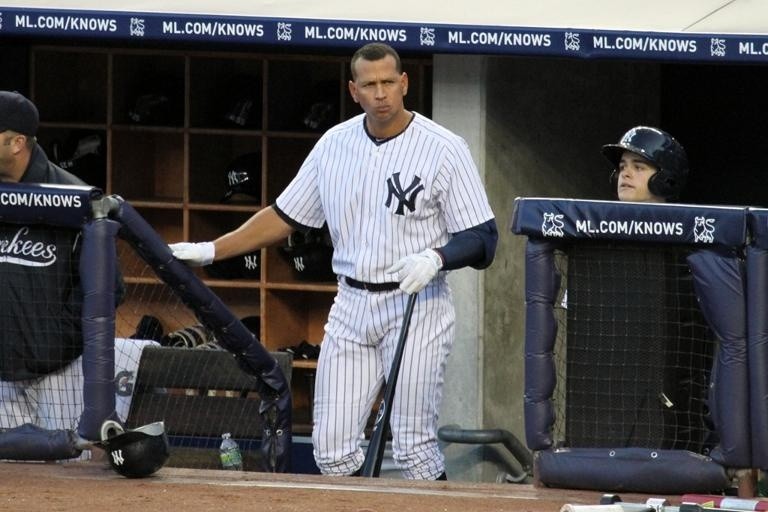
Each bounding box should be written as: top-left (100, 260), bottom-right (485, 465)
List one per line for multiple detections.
top-left (123, 344), bottom-right (293, 472)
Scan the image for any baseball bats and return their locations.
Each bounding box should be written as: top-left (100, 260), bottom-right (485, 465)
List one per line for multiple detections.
top-left (362, 293), bottom-right (417, 478)
top-left (557, 493), bottom-right (768, 511)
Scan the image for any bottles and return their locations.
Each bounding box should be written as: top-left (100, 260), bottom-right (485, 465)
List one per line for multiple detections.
top-left (219, 432), bottom-right (242, 471)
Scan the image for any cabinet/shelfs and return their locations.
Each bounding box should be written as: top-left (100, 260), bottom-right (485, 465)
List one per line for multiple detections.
top-left (0, 31), bottom-right (432, 441)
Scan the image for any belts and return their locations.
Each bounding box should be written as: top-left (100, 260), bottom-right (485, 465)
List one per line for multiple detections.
top-left (345, 276), bottom-right (401, 293)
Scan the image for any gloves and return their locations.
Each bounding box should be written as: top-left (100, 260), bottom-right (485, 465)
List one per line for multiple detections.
top-left (385, 248), bottom-right (444, 296)
top-left (167, 241), bottom-right (216, 268)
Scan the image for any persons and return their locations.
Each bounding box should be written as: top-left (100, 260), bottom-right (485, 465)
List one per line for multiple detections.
top-left (166, 42), bottom-right (498, 481)
top-left (0, 90), bottom-right (125, 463)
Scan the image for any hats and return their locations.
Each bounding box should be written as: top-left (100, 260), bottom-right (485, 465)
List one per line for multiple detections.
top-left (212, 72), bottom-right (263, 128)
top-left (221, 150), bottom-right (262, 205)
top-left (126, 90), bottom-right (174, 126)
top-left (53, 128), bottom-right (105, 186)
top-left (276, 228), bottom-right (337, 282)
top-left (0, 90), bottom-right (40, 137)
top-left (203, 250), bottom-right (261, 280)
top-left (297, 83), bottom-right (341, 131)
top-left (129, 314), bottom-right (260, 348)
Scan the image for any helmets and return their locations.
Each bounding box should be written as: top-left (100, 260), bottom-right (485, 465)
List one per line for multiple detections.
top-left (100, 419), bottom-right (170, 479)
